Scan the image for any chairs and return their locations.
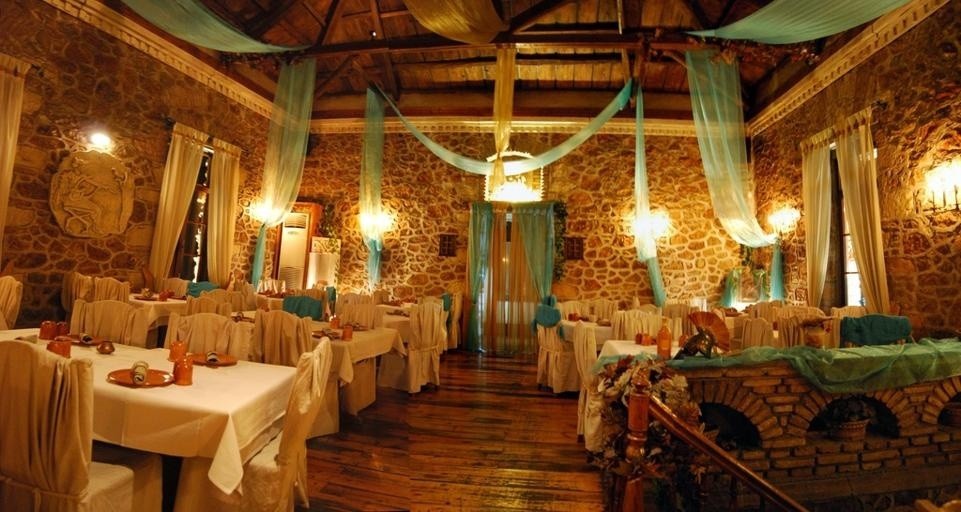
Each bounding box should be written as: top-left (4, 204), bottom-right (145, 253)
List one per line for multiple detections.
top-left (170, 336), bottom-right (336, 512)
top-left (0, 275), bottom-right (23, 330)
top-left (62, 271), bottom-right (465, 413)
top-left (1, 340), bottom-right (133, 509)
top-left (533, 286), bottom-right (911, 461)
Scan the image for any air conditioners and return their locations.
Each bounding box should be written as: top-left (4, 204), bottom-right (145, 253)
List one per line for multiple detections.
top-left (276, 211), bottom-right (310, 291)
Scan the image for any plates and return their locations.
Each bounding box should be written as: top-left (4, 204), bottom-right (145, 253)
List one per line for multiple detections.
top-left (62, 335), bottom-right (107, 346)
top-left (186, 352), bottom-right (238, 366)
top-left (343, 323), bottom-right (367, 331)
top-left (108, 369), bottom-right (174, 387)
top-left (133, 294), bottom-right (158, 301)
top-left (310, 331), bottom-right (340, 339)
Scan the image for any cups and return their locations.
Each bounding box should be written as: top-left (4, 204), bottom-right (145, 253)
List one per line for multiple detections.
top-left (168, 340), bottom-right (187, 363)
top-left (678, 335), bottom-right (689, 347)
top-left (567, 311), bottom-right (597, 323)
top-left (172, 358), bottom-right (194, 386)
top-left (39, 320), bottom-right (72, 360)
top-left (95, 340), bottom-right (115, 355)
top-left (342, 326), bottom-right (353, 340)
top-left (331, 318), bottom-right (340, 328)
top-left (635, 333), bottom-right (652, 346)
top-left (160, 288), bottom-right (175, 301)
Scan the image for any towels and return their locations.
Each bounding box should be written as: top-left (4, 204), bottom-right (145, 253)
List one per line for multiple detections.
top-left (14, 333), bottom-right (39, 346)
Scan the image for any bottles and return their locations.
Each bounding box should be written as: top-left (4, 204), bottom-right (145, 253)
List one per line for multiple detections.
top-left (656, 317), bottom-right (672, 359)
